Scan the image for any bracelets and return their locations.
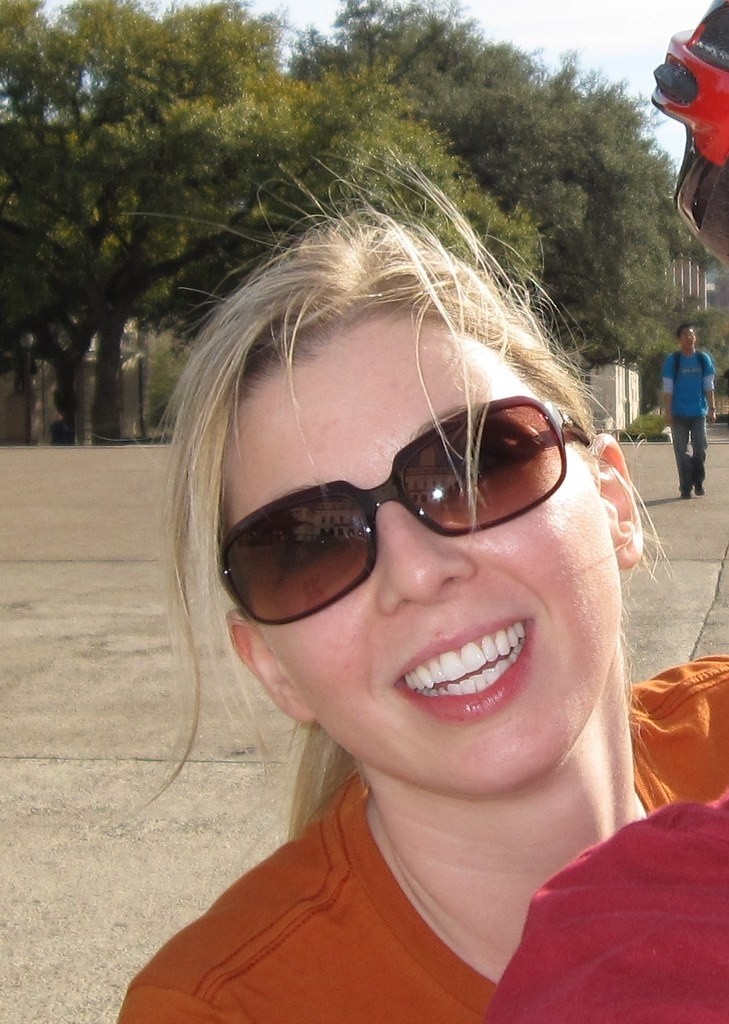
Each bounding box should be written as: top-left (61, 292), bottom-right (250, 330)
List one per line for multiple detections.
top-left (708, 406), bottom-right (717, 411)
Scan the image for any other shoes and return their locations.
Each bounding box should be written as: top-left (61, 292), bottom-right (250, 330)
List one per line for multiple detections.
top-left (681, 490), bottom-right (691, 500)
top-left (692, 480), bottom-right (705, 495)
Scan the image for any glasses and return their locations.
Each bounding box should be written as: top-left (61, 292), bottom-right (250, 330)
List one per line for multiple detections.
top-left (216, 395), bottom-right (593, 626)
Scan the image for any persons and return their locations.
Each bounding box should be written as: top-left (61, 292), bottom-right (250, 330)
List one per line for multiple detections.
top-left (454, 0), bottom-right (729, 1024)
top-left (108, 142), bottom-right (729, 1024)
top-left (661, 319), bottom-right (720, 500)
